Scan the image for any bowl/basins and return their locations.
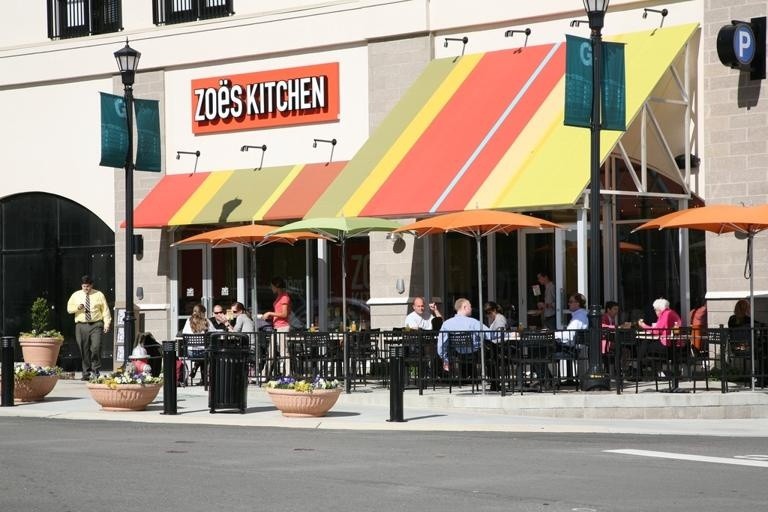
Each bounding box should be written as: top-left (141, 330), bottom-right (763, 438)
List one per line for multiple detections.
top-left (509, 327), bottom-right (517, 331)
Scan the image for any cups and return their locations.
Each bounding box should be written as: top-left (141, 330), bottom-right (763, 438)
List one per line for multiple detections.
top-left (529, 326), bottom-right (536, 333)
top-left (256, 313), bottom-right (262, 320)
top-left (540, 329), bottom-right (548, 334)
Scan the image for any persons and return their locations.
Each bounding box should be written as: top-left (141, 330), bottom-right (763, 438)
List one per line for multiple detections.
top-left (536, 270), bottom-right (556, 330)
top-left (601, 295), bottom-right (707, 381)
top-left (483, 302), bottom-right (508, 330)
top-left (66, 274), bottom-right (113, 380)
top-left (437, 298), bottom-right (525, 385)
top-left (728, 299), bottom-right (768, 356)
top-left (261, 278), bottom-right (291, 377)
top-left (405, 297), bottom-right (444, 378)
top-left (181, 301), bottom-right (256, 385)
top-left (533, 293), bottom-right (589, 383)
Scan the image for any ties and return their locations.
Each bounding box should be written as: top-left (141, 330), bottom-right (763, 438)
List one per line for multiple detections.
top-left (85, 293), bottom-right (91, 321)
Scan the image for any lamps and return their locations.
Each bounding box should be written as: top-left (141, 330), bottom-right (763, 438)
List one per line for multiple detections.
top-left (444, 36), bottom-right (468, 48)
top-left (505, 28), bottom-right (531, 37)
top-left (643, 8), bottom-right (668, 19)
top-left (240, 144), bottom-right (267, 152)
top-left (570, 19), bottom-right (590, 27)
top-left (176, 151), bottom-right (200, 160)
top-left (313, 138), bottom-right (336, 148)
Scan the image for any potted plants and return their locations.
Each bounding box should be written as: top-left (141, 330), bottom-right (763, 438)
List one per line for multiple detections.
top-left (17, 297), bottom-right (65, 367)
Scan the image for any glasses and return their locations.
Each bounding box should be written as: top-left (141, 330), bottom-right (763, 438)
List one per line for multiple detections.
top-left (233, 310), bottom-right (239, 313)
top-left (215, 312), bottom-right (222, 314)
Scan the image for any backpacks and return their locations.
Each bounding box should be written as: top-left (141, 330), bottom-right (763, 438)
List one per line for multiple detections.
top-left (175, 356), bottom-right (189, 387)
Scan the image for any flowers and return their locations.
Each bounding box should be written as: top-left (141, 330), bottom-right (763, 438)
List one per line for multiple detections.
top-left (14, 362), bottom-right (62, 378)
top-left (88, 363), bottom-right (164, 389)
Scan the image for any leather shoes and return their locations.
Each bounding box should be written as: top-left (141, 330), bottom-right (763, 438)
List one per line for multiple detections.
top-left (81, 375), bottom-right (89, 381)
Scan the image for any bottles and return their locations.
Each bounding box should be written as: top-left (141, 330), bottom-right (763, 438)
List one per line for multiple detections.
top-left (672, 321), bottom-right (680, 337)
top-left (518, 322), bottom-right (524, 331)
top-left (404, 323), bottom-right (410, 333)
top-left (307, 323), bottom-right (319, 333)
top-left (329, 319), bottom-right (365, 332)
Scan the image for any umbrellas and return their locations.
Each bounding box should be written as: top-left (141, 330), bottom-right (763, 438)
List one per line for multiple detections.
top-left (631, 202), bottom-right (768, 390)
top-left (392, 200), bottom-right (569, 396)
top-left (264, 212), bottom-right (417, 394)
top-left (169, 216), bottom-right (337, 386)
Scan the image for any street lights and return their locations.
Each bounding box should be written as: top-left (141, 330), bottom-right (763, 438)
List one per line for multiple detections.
top-left (582, 0), bottom-right (613, 391)
top-left (112, 35), bottom-right (145, 376)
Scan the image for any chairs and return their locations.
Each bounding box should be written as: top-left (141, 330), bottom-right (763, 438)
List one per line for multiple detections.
top-left (177, 325), bottom-right (768, 398)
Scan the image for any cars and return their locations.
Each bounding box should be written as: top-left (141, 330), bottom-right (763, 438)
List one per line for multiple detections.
top-left (294, 299), bottom-right (371, 331)
top-left (215, 286), bottom-right (305, 317)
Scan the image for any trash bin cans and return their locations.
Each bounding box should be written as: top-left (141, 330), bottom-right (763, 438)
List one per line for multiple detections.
top-left (206, 330), bottom-right (250, 414)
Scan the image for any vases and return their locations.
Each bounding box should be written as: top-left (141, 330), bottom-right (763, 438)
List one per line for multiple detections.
top-left (86, 381), bottom-right (162, 411)
top-left (0, 376), bottom-right (61, 402)
top-left (265, 388), bottom-right (342, 417)
